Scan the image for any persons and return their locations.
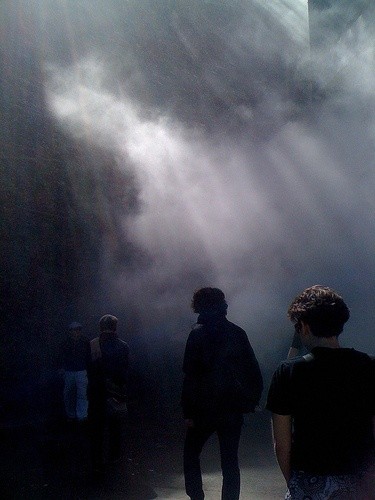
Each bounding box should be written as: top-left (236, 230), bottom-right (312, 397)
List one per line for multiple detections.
top-left (62, 313), bottom-right (132, 469)
top-left (266, 283), bottom-right (374, 500)
top-left (178, 286), bottom-right (265, 500)
